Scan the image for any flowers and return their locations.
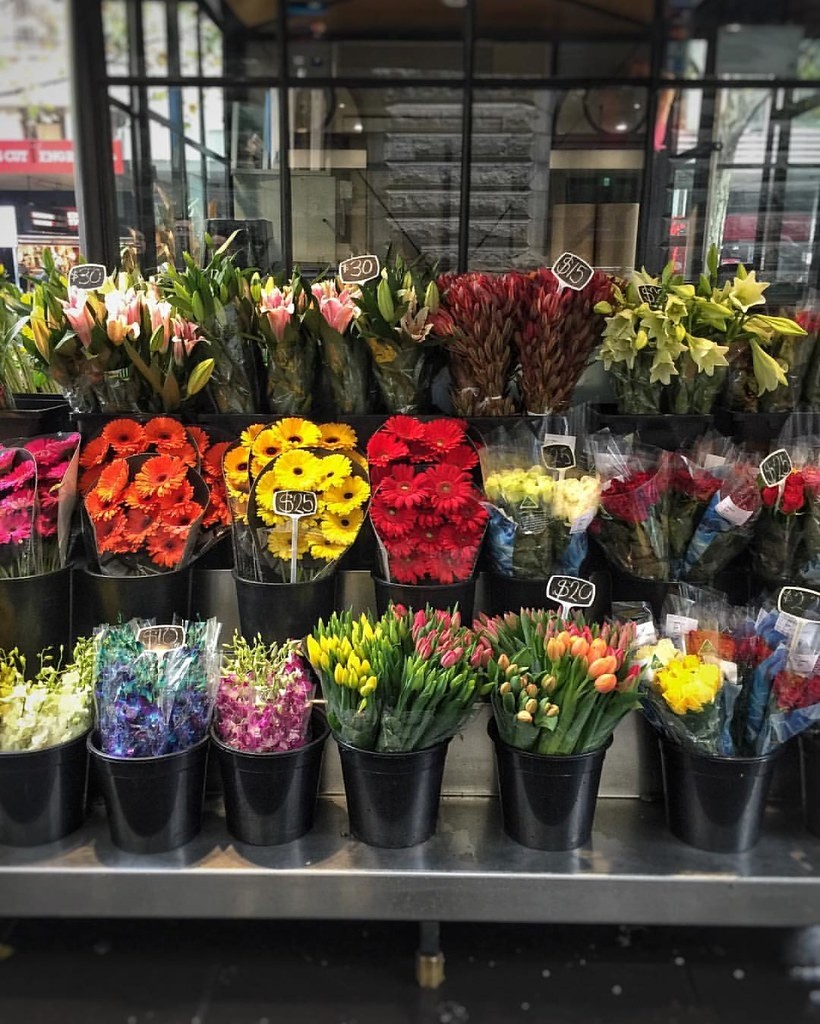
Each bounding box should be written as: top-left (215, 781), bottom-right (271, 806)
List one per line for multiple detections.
top-left (0, 241), bottom-right (819, 419)
top-left (0, 592), bottom-right (820, 758)
top-left (0, 415), bottom-right (820, 591)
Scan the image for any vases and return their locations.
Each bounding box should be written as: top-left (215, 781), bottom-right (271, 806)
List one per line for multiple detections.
top-left (231, 565), bottom-right (336, 650)
top-left (748, 569), bottom-right (806, 591)
top-left (86, 561), bottom-right (195, 627)
top-left (0, 560), bottom-right (76, 682)
top-left (584, 401), bottom-right (708, 450)
top-left (370, 570), bottom-right (481, 628)
top-left (0, 398), bottom-right (71, 441)
top-left (612, 566), bottom-right (708, 611)
top-left (487, 565), bottom-right (592, 616)
top-left (714, 401), bottom-right (790, 449)
top-left (3, 728), bottom-right (820, 854)
